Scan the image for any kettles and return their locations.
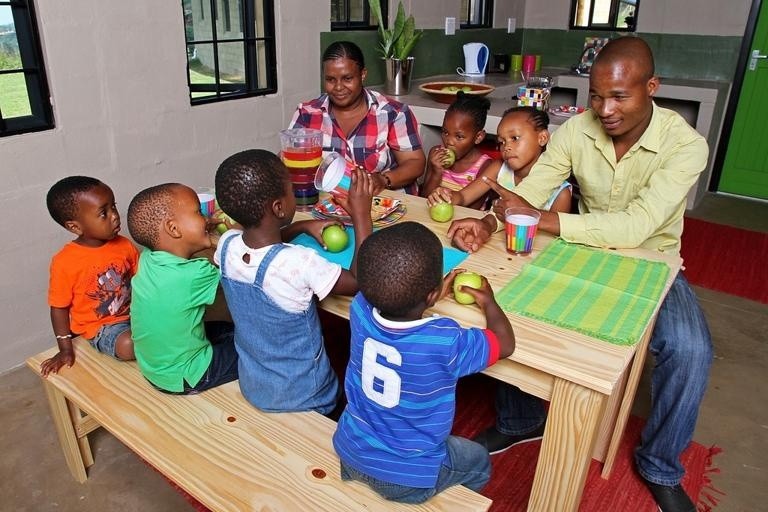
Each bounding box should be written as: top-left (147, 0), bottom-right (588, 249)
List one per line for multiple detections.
top-left (461, 43), bottom-right (490, 77)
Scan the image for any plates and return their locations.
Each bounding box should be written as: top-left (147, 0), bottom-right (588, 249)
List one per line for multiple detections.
top-left (549, 106), bottom-right (589, 118)
top-left (313, 193), bottom-right (408, 229)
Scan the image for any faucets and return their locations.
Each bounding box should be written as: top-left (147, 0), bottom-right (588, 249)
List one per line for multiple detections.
top-left (571, 45), bottom-right (593, 75)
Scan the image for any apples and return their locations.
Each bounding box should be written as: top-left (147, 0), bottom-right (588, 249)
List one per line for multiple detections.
top-left (321, 226), bottom-right (350, 253)
top-left (453, 272), bottom-right (481, 305)
top-left (431, 200), bottom-right (453, 222)
top-left (440, 86), bottom-right (473, 93)
top-left (217, 213), bottom-right (235, 233)
top-left (439, 149), bottom-right (457, 169)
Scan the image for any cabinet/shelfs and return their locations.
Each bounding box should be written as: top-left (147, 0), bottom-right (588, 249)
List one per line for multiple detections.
top-left (207, 187), bottom-right (683, 512)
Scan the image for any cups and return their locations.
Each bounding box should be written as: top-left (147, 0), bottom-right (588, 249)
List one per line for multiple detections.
top-left (279, 127), bottom-right (325, 208)
top-left (495, 53), bottom-right (509, 73)
top-left (523, 55), bottom-right (536, 73)
top-left (504, 207), bottom-right (541, 256)
top-left (195, 192), bottom-right (216, 218)
top-left (533, 56), bottom-right (542, 73)
top-left (510, 55), bottom-right (523, 72)
top-left (313, 152), bottom-right (361, 201)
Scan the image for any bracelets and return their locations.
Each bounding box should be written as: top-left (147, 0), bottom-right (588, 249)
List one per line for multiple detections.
top-left (377, 171), bottom-right (392, 189)
top-left (54, 335), bottom-right (73, 341)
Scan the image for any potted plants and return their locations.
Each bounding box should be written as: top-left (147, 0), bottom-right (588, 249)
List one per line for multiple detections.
top-left (366, 0), bottom-right (424, 96)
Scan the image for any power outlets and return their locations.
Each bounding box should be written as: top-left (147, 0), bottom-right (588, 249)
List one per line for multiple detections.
top-left (508, 18), bottom-right (517, 34)
top-left (446, 18), bottom-right (456, 35)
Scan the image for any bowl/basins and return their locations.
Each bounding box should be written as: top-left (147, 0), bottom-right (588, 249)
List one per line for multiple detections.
top-left (420, 81), bottom-right (495, 103)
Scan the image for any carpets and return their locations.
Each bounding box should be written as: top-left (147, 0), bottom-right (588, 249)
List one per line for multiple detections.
top-left (127, 379), bottom-right (724, 512)
top-left (680, 217), bottom-right (768, 305)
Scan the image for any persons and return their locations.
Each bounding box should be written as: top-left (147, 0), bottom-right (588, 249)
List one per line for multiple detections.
top-left (285, 40), bottom-right (425, 193)
top-left (332, 221), bottom-right (515, 505)
top-left (39, 176), bottom-right (140, 377)
top-left (214, 149), bottom-right (375, 414)
top-left (427, 108), bottom-right (574, 213)
top-left (447, 36), bottom-right (714, 512)
top-left (419, 91), bottom-right (495, 210)
top-left (127, 184), bottom-right (239, 395)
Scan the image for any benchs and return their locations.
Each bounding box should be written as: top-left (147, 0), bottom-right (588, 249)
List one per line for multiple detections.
top-left (26, 333), bottom-right (494, 512)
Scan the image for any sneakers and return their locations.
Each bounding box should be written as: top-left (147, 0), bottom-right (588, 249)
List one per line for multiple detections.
top-left (647, 482), bottom-right (695, 511)
top-left (475, 425), bottom-right (544, 455)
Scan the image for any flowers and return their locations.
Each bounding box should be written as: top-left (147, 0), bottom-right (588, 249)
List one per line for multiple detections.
top-left (624, 11), bottom-right (634, 28)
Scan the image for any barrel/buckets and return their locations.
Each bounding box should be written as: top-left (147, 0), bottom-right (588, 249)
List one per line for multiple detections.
top-left (383, 57), bottom-right (416, 95)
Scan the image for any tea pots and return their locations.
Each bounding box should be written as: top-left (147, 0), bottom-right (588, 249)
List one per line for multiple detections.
top-left (575, 45), bottom-right (598, 77)
